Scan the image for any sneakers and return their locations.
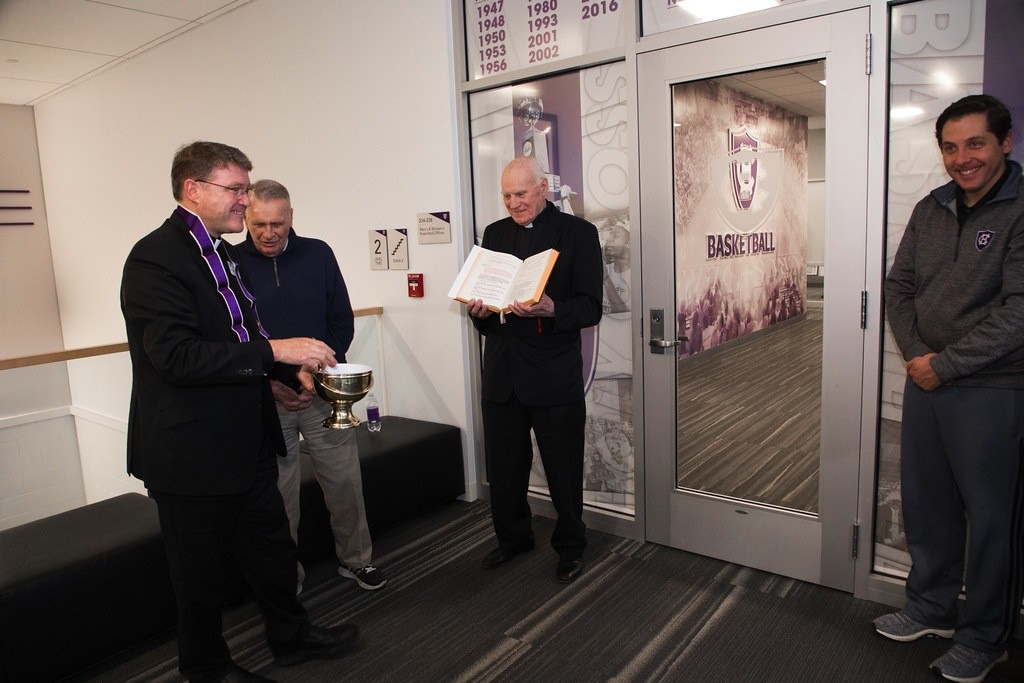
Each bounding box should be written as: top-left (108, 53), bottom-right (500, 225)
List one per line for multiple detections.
top-left (338, 561), bottom-right (387, 589)
top-left (929, 642), bottom-right (1009, 683)
top-left (871, 611), bottom-right (954, 642)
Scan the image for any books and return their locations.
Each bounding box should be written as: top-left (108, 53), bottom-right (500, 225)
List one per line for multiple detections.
top-left (447, 244), bottom-right (559, 311)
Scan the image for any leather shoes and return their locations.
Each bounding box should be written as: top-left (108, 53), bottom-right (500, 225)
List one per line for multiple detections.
top-left (271, 624), bottom-right (360, 668)
top-left (482, 534), bottom-right (535, 569)
top-left (223, 665), bottom-right (280, 683)
top-left (557, 556), bottom-right (583, 584)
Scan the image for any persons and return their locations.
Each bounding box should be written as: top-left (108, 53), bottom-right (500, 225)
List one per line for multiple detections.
top-left (120, 141), bottom-right (362, 682)
top-left (467, 157), bottom-right (603, 583)
top-left (235, 178), bottom-right (387, 598)
top-left (873, 95), bottom-right (1024, 683)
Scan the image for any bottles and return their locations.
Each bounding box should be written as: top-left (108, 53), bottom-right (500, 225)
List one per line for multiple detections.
top-left (366, 393), bottom-right (381, 432)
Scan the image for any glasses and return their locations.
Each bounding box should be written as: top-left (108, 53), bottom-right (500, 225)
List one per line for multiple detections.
top-left (193, 179), bottom-right (255, 200)
